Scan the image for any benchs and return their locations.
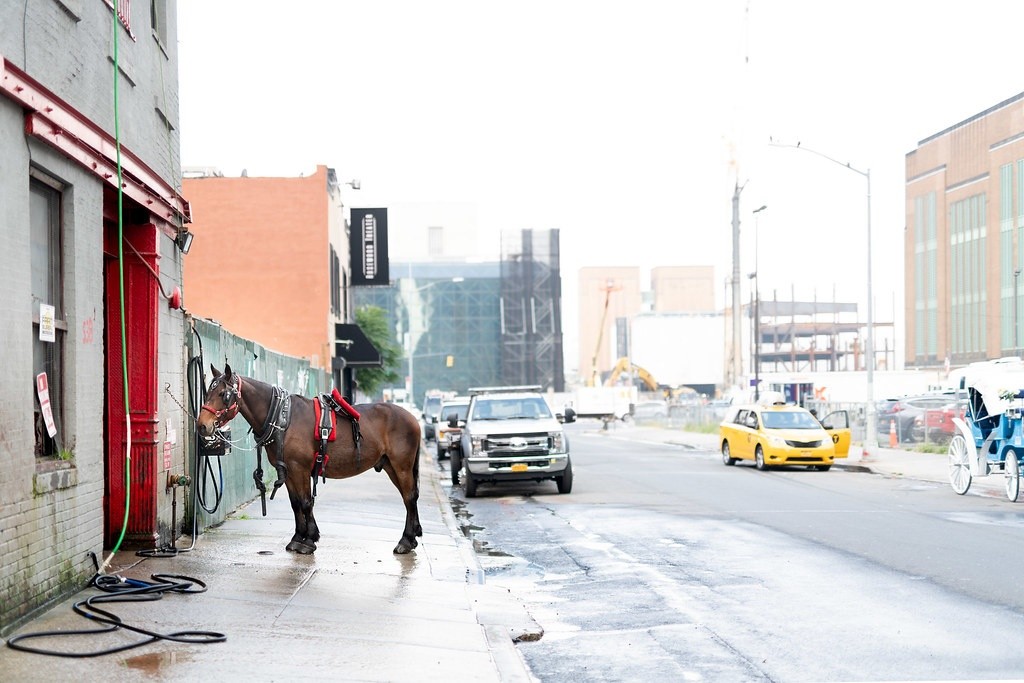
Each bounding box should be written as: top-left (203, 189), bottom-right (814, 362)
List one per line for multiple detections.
top-left (1009, 389), bottom-right (1024, 415)
top-left (975, 411), bottom-right (1014, 440)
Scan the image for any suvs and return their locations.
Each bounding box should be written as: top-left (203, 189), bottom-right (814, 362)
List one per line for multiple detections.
top-left (447, 384), bottom-right (577, 499)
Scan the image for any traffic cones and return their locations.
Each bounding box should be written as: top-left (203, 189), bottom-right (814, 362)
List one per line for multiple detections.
top-left (888, 418), bottom-right (900, 449)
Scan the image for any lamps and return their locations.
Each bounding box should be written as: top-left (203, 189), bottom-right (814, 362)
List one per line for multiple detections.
top-left (330, 178), bottom-right (360, 190)
top-left (175, 231), bottom-right (194, 253)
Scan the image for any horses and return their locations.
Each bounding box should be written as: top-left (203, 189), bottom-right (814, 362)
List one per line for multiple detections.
top-left (199, 363), bottom-right (422, 555)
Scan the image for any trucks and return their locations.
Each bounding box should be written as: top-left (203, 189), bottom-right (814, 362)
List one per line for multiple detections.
top-left (539, 384), bottom-right (670, 423)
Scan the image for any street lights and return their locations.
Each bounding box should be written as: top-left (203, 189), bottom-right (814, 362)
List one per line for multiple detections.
top-left (1014, 269), bottom-right (1021, 351)
top-left (746, 204), bottom-right (769, 401)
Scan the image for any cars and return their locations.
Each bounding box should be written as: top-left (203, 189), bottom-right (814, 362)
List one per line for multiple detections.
top-left (385, 389), bottom-right (472, 460)
top-left (717, 392), bottom-right (851, 472)
top-left (854, 367), bottom-right (969, 446)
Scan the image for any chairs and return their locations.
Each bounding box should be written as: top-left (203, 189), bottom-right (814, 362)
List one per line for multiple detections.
top-left (524, 404), bottom-right (535, 413)
top-left (480, 405), bottom-right (491, 416)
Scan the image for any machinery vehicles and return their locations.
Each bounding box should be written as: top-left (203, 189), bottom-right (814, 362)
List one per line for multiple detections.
top-left (584, 277), bottom-right (711, 419)
